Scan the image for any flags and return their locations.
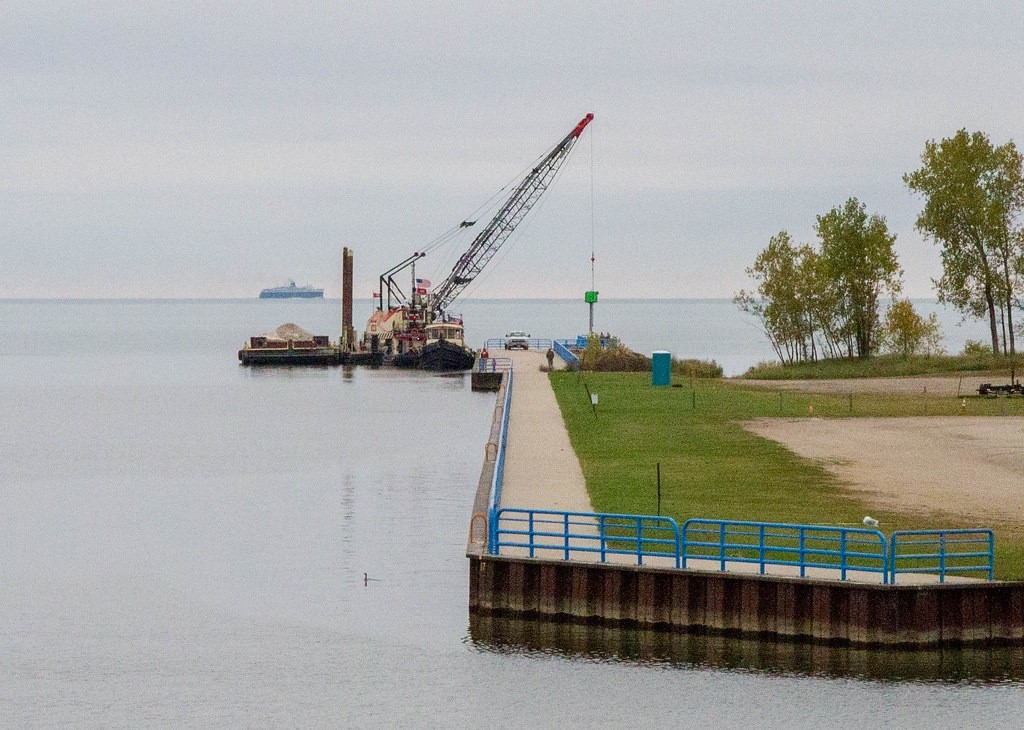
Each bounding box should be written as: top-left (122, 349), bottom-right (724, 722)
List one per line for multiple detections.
top-left (416, 278), bottom-right (431, 288)
top-left (374, 292), bottom-right (381, 298)
top-left (418, 288), bottom-right (426, 295)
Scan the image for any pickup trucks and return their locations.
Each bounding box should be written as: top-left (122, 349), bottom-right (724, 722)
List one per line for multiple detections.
top-left (504, 331), bottom-right (530, 350)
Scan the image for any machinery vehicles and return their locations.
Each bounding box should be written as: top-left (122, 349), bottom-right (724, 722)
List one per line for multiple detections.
top-left (363, 112), bottom-right (598, 352)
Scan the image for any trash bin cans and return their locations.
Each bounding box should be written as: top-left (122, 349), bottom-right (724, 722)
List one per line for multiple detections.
top-left (651, 350), bottom-right (672, 385)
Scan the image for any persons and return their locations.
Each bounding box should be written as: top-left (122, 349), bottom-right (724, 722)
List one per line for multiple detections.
top-left (492, 358), bottom-right (496, 371)
top-left (546, 349), bottom-right (554, 367)
top-left (481, 347), bottom-right (488, 371)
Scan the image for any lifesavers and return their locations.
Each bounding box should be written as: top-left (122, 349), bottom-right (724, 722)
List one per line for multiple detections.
top-left (411, 328), bottom-right (419, 336)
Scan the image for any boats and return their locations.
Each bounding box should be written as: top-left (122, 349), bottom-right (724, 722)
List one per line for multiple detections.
top-left (418, 319), bottom-right (476, 374)
top-left (258, 279), bottom-right (324, 300)
